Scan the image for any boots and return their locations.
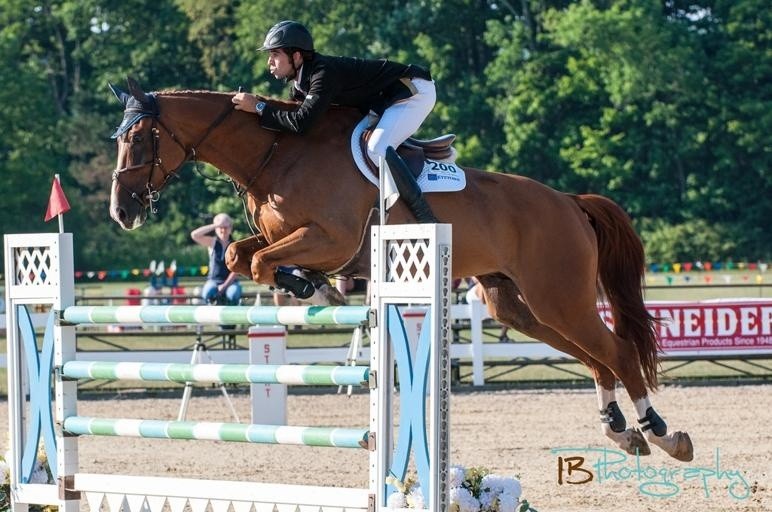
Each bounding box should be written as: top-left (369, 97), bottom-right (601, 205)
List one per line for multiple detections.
top-left (384, 149), bottom-right (439, 245)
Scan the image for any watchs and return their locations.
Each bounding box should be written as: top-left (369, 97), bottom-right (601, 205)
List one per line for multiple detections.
top-left (255, 102), bottom-right (266, 116)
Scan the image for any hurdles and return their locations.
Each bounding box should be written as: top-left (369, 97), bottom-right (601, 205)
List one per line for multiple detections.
top-left (3, 224), bottom-right (454, 512)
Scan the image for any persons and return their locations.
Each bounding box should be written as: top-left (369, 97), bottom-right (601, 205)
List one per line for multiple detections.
top-left (464, 275), bottom-right (516, 344)
top-left (335, 275), bottom-right (364, 295)
top-left (272, 263), bottom-right (304, 334)
top-left (231, 20), bottom-right (441, 226)
top-left (190, 212), bottom-right (242, 338)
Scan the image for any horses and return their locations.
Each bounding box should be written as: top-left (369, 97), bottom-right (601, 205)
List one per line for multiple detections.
top-left (107, 73), bottom-right (695, 464)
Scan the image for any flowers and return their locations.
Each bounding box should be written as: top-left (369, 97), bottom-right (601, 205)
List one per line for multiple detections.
top-left (384, 462), bottom-right (535, 511)
top-left (1, 436), bottom-right (59, 512)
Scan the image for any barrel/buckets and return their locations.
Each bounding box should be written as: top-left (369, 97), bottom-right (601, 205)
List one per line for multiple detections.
top-left (127, 288), bottom-right (141, 305)
top-left (173, 286), bottom-right (187, 305)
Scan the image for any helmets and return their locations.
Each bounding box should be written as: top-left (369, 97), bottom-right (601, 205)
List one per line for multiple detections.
top-left (257, 20), bottom-right (314, 53)
